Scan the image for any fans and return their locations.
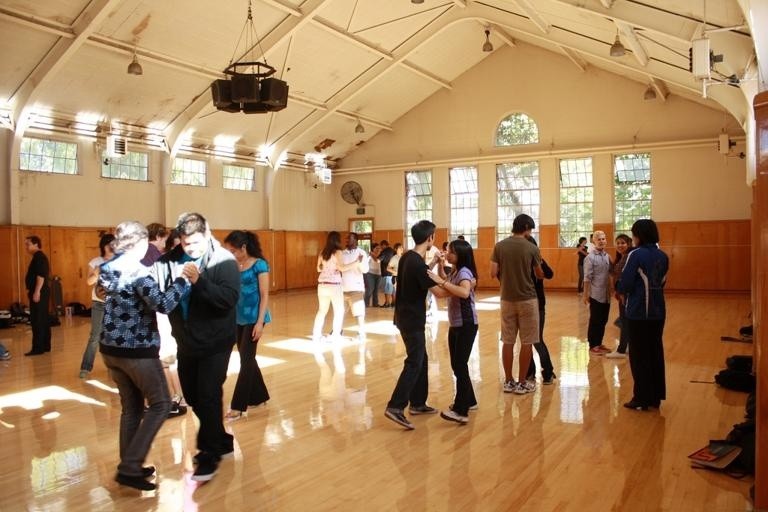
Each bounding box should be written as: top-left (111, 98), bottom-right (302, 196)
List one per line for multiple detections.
top-left (341, 181), bottom-right (366, 215)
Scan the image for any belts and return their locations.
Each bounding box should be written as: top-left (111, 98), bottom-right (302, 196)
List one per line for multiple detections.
top-left (318, 281), bottom-right (340, 284)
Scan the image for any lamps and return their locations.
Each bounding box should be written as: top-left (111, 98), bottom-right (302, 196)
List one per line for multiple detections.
top-left (128, 36), bottom-right (143, 74)
top-left (483, 30), bottom-right (493, 52)
top-left (355, 115), bottom-right (364, 133)
top-left (610, 27), bottom-right (625, 57)
top-left (209, 2), bottom-right (289, 114)
top-left (644, 84), bottom-right (656, 99)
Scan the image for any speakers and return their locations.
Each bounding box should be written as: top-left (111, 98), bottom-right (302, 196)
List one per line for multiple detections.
top-left (210, 74), bottom-right (289, 114)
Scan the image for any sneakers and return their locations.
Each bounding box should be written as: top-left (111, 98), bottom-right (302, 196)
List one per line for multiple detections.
top-left (373, 303), bottom-right (395, 308)
top-left (167, 404), bottom-right (187, 418)
top-left (503, 372), bottom-right (554, 394)
top-left (589, 345), bottom-right (626, 358)
top-left (191, 447), bottom-right (234, 481)
top-left (385, 404), bottom-right (478, 429)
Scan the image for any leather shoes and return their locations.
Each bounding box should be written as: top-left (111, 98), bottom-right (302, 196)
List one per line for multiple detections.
top-left (80, 369), bottom-right (88, 378)
top-left (24, 348), bottom-right (51, 356)
top-left (115, 466), bottom-right (155, 490)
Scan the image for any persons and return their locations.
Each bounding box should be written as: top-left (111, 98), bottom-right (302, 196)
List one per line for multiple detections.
top-left (24, 236), bottom-right (52, 356)
top-left (490, 214), bottom-right (670, 412)
top-left (312, 220), bottom-right (479, 430)
top-left (80, 212), bottom-right (270, 490)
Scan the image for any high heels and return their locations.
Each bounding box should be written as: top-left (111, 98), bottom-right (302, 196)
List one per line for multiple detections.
top-left (224, 401), bottom-right (267, 420)
top-left (623, 396), bottom-right (660, 411)
